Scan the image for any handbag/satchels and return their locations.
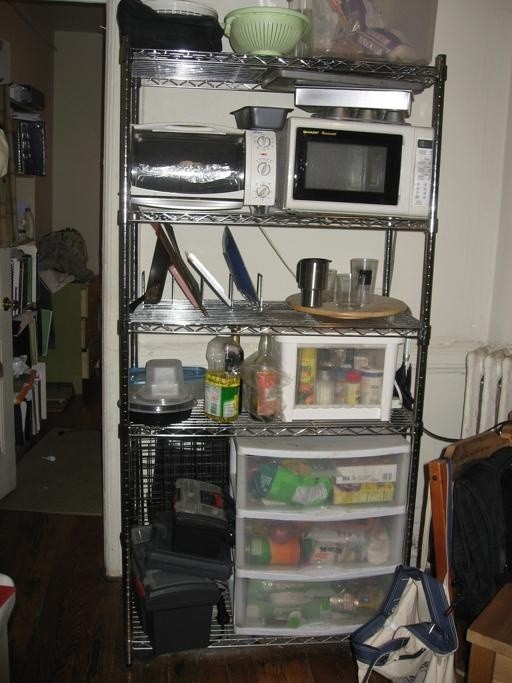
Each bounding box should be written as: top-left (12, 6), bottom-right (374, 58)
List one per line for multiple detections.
top-left (352, 564), bottom-right (460, 683)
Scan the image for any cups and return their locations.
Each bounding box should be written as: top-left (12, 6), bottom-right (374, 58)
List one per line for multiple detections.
top-left (323, 256), bottom-right (380, 311)
top-left (329, 587), bottom-right (384, 617)
top-left (295, 257), bottom-right (331, 309)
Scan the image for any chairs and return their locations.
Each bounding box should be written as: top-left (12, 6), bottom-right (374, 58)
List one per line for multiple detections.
top-left (427, 422), bottom-right (511, 682)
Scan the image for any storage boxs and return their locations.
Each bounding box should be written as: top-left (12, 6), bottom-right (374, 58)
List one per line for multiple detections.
top-left (269, 332), bottom-right (405, 425)
top-left (229, 427), bottom-right (412, 637)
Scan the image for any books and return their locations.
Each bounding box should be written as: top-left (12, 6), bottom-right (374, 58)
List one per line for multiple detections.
top-left (9, 244), bottom-right (53, 448)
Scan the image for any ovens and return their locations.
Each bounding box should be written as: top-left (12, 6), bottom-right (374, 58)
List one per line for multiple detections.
top-left (125, 120), bottom-right (277, 212)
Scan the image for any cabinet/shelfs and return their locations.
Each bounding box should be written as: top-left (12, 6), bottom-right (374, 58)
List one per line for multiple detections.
top-left (1, 86), bottom-right (46, 498)
top-left (49, 275), bottom-right (97, 378)
top-left (115, 36), bottom-right (444, 672)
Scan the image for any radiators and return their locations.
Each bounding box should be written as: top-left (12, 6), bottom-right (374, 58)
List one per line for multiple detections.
top-left (461, 347), bottom-right (512, 440)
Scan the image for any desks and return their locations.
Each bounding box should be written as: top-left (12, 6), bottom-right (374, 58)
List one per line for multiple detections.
top-left (464, 577), bottom-right (511, 683)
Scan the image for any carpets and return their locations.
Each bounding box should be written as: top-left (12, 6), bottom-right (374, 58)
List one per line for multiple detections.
top-left (1, 426), bottom-right (102, 518)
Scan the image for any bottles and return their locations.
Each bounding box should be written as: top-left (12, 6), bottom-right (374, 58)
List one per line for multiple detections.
top-left (204, 327), bottom-right (280, 423)
top-left (16, 205), bottom-right (35, 241)
top-left (313, 358), bottom-right (362, 407)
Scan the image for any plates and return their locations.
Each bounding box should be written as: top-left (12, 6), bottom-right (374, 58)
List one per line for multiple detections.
top-left (185, 249), bottom-right (234, 308)
top-left (220, 224), bottom-right (260, 308)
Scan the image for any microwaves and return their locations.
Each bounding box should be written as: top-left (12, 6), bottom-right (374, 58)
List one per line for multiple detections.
top-left (276, 115), bottom-right (435, 224)
